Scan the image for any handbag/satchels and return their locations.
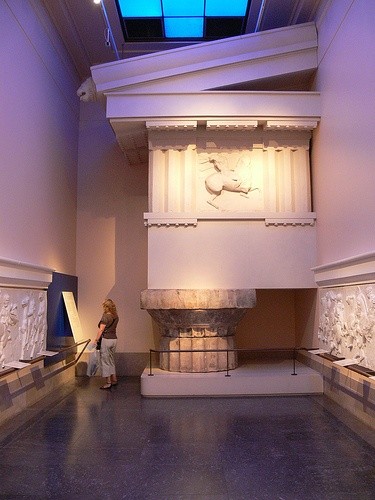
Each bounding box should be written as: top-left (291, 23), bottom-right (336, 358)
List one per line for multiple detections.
top-left (96, 337), bottom-right (102, 350)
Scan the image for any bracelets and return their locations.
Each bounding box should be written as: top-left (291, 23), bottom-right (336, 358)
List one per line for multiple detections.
top-left (95, 339), bottom-right (100, 342)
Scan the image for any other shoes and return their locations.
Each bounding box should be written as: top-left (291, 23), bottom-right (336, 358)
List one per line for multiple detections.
top-left (112, 381), bottom-right (118, 386)
top-left (100, 382), bottom-right (112, 389)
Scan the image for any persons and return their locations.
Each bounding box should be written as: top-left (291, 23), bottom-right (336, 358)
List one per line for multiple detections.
top-left (93, 298), bottom-right (119, 391)
